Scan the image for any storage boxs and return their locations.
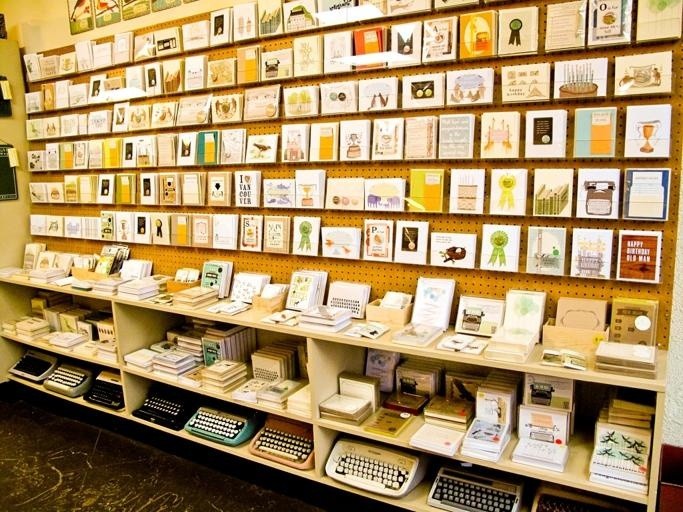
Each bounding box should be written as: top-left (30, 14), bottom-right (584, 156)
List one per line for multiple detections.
top-left (364, 297), bottom-right (414, 327)
top-left (250, 291), bottom-right (286, 314)
top-left (68, 265), bottom-right (119, 282)
top-left (541, 317), bottom-right (611, 351)
top-left (165, 279), bottom-right (201, 294)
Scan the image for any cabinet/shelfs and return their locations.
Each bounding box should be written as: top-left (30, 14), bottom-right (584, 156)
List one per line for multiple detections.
top-left (0, 275), bottom-right (671, 512)
top-left (25, 0), bottom-right (683, 351)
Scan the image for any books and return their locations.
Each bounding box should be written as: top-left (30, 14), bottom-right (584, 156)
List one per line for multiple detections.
top-left (2, 243), bottom-right (658, 492)
top-left (22, 0), bottom-right (682, 287)
